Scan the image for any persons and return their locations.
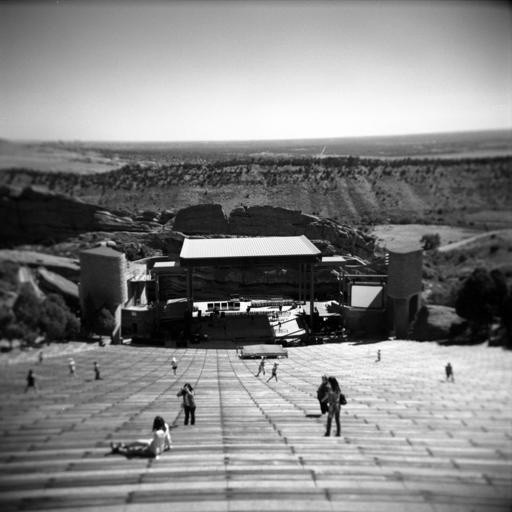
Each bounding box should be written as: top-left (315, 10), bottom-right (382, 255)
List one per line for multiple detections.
top-left (374, 348), bottom-right (382, 363)
top-left (253, 356), bottom-right (267, 378)
top-left (176, 381), bottom-right (198, 426)
top-left (444, 362), bottom-right (454, 383)
top-left (265, 362), bottom-right (280, 383)
top-left (109, 415), bottom-right (172, 460)
top-left (321, 376), bottom-right (346, 437)
top-left (38, 351), bottom-right (44, 363)
top-left (316, 374), bottom-right (348, 416)
top-left (171, 357), bottom-right (178, 377)
top-left (22, 369), bottom-right (39, 394)
top-left (93, 360), bottom-right (105, 381)
top-left (65, 357), bottom-right (78, 377)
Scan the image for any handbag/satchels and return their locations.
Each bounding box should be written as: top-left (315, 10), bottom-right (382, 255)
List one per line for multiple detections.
top-left (341, 394), bottom-right (347, 406)
